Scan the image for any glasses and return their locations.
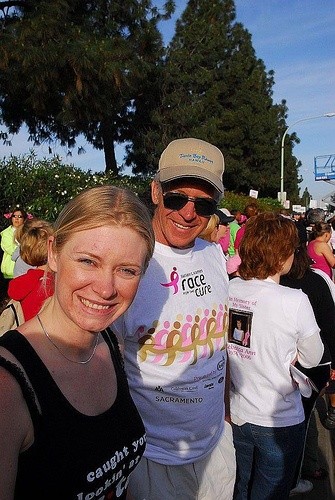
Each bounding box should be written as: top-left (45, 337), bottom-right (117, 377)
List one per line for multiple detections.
top-left (215, 224), bottom-right (219, 229)
top-left (163, 192), bottom-right (217, 217)
top-left (12, 215), bottom-right (22, 218)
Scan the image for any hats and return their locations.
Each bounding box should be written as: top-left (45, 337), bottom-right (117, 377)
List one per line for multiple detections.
top-left (215, 210), bottom-right (236, 224)
top-left (157, 138), bottom-right (225, 194)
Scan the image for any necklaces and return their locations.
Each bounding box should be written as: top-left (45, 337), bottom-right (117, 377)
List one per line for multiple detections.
top-left (36, 312), bottom-right (101, 364)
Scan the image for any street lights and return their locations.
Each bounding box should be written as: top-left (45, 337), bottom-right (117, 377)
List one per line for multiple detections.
top-left (277, 113), bottom-right (335, 207)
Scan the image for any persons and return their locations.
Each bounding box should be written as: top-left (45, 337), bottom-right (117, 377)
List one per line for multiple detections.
top-left (0, 186), bottom-right (155, 500)
top-left (109, 137), bottom-right (237, 500)
top-left (233, 319), bottom-right (244, 342)
top-left (227, 213), bottom-right (325, 500)
top-left (0, 206), bottom-right (335, 495)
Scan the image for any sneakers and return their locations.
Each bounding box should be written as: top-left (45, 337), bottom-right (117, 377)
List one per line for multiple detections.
top-left (289, 479), bottom-right (313, 495)
